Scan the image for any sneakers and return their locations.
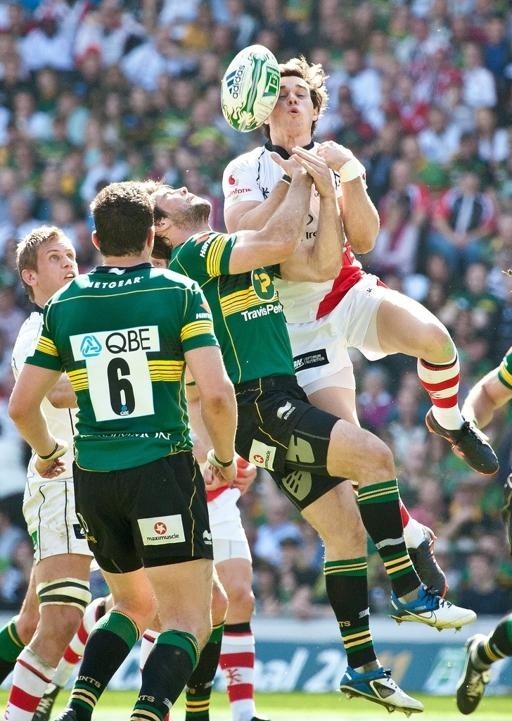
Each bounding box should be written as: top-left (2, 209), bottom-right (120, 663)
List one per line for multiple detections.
top-left (404, 525), bottom-right (447, 603)
top-left (29, 687), bottom-right (60, 721)
top-left (455, 631), bottom-right (496, 716)
top-left (424, 405), bottom-right (501, 477)
top-left (389, 582), bottom-right (478, 633)
top-left (335, 663), bottom-right (426, 719)
top-left (54, 707), bottom-right (80, 721)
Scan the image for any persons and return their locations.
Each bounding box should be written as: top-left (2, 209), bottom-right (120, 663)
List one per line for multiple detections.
top-left (152, 169), bottom-right (477, 716)
top-left (358, 374), bottom-right (508, 594)
top-left (221, 3), bottom-right (512, 54)
top-left (5, 562), bottom-right (229, 716)
top-left (223, 57), bottom-right (498, 597)
top-left (10, 181), bottom-right (237, 721)
top-left (2, 184), bottom-right (112, 352)
top-left (455, 265), bottom-right (511, 716)
top-left (363, 163), bottom-right (512, 373)
top-left (1, 1), bottom-right (222, 180)
top-left (235, 483), bottom-right (334, 621)
top-left (4, 225), bottom-right (116, 721)
top-left (138, 237), bottom-right (257, 720)
top-left (318, 56), bottom-right (511, 164)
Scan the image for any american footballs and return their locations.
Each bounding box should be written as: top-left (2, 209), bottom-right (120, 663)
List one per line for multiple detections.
top-left (221, 44), bottom-right (281, 133)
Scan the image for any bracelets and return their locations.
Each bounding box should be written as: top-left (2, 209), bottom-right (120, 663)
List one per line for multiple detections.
top-left (280, 173), bottom-right (291, 183)
top-left (212, 454), bottom-right (234, 467)
top-left (37, 443), bottom-right (58, 461)
